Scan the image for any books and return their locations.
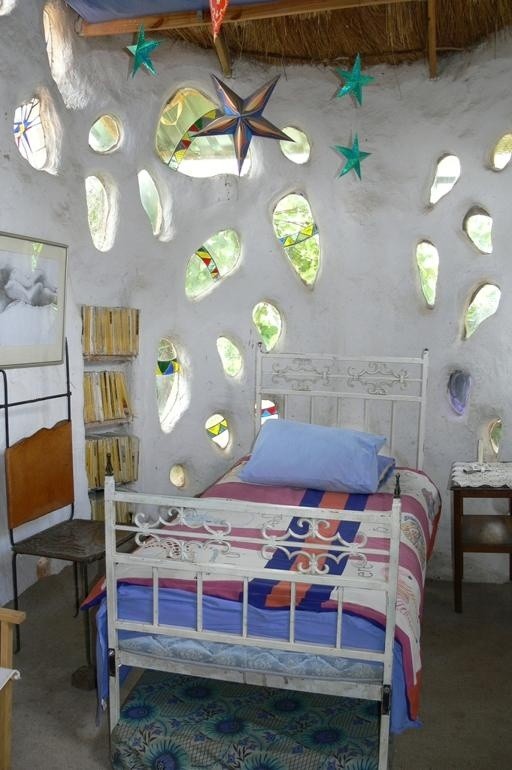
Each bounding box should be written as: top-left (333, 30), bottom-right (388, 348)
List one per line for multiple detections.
top-left (79, 303), bottom-right (142, 524)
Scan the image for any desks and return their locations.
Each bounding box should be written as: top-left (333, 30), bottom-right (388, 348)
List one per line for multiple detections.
top-left (447, 460), bottom-right (511, 615)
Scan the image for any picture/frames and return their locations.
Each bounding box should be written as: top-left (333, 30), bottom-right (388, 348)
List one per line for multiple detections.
top-left (0, 230), bottom-right (68, 370)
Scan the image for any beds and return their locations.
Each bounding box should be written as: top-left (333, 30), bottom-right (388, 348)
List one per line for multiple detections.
top-left (100, 342), bottom-right (431, 769)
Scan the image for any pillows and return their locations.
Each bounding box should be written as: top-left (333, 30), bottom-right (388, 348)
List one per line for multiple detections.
top-left (232, 419), bottom-right (396, 494)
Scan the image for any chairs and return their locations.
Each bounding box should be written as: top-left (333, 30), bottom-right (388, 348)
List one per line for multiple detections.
top-left (3, 419), bottom-right (138, 669)
